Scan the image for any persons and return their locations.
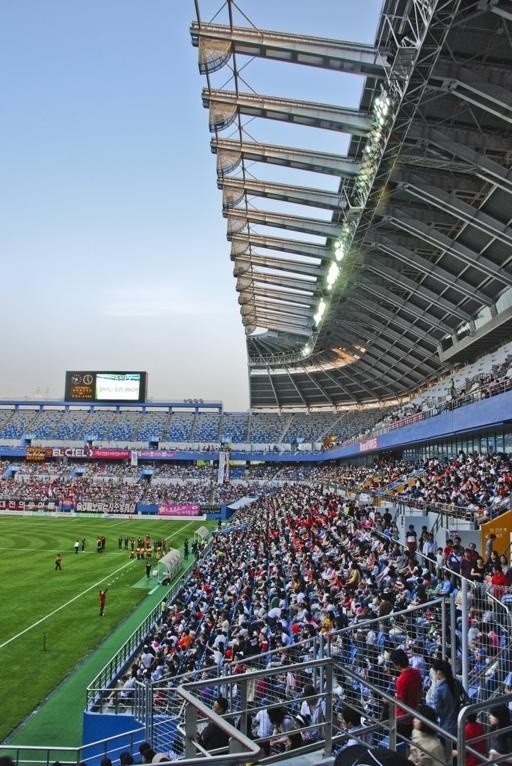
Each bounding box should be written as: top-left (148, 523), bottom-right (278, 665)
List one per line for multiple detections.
top-left (0, 755), bottom-right (17, 766)
top-left (94, 533), bottom-right (202, 766)
top-left (81, 537), bottom-right (86, 552)
top-left (54, 553), bottom-right (63, 571)
top-left (1, 369), bottom-right (511, 517)
top-left (202, 517), bottom-right (512, 765)
top-left (74, 540), bottom-right (81, 554)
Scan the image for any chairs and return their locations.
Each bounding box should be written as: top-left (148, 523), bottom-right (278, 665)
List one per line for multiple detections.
top-left (2, 405), bottom-right (354, 445)
top-left (356, 336), bottom-right (510, 445)
top-left (340, 450), bottom-right (512, 507)
top-left (1, 453), bottom-right (338, 507)
top-left (115, 507), bottom-right (512, 765)
